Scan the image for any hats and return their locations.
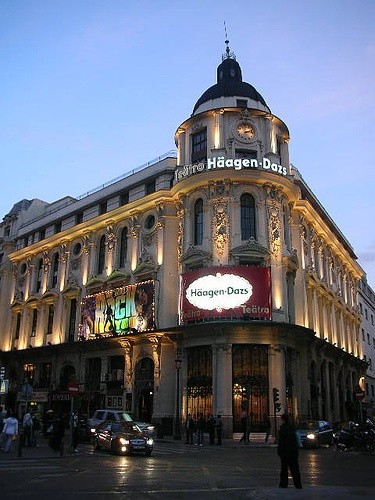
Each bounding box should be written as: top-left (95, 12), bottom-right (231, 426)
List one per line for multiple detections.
top-left (280, 413), bottom-right (289, 421)
top-left (46, 409), bottom-right (53, 414)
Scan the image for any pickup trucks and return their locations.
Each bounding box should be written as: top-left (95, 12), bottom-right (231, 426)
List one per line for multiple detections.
top-left (73, 409), bottom-right (154, 437)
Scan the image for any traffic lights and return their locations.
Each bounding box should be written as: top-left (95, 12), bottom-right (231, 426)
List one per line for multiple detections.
top-left (275, 402), bottom-right (280, 412)
top-left (273, 388), bottom-right (279, 402)
top-left (241, 384), bottom-right (250, 400)
top-left (285, 387), bottom-right (292, 399)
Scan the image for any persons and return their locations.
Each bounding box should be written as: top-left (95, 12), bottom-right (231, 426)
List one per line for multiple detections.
top-left (195, 416), bottom-right (205, 447)
top-left (0, 404), bottom-right (81, 459)
top-left (274, 412), bottom-right (303, 490)
top-left (239, 409), bottom-right (252, 443)
top-left (263, 415), bottom-right (272, 443)
top-left (184, 414), bottom-right (195, 445)
top-left (212, 415), bottom-right (222, 445)
top-left (206, 414), bottom-right (215, 445)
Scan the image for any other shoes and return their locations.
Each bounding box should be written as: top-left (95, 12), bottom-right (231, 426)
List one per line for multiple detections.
top-left (194, 442), bottom-right (204, 447)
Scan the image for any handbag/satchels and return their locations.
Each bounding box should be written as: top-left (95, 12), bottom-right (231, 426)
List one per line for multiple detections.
top-left (12, 434), bottom-right (17, 441)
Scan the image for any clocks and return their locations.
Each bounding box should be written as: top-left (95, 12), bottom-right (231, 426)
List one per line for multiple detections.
top-left (232, 119), bottom-right (260, 144)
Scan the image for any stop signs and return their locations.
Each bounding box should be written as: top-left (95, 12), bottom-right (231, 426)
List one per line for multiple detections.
top-left (68, 382), bottom-right (79, 396)
top-left (355, 389), bottom-right (365, 401)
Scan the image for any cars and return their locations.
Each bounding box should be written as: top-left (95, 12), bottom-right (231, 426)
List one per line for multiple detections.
top-left (92, 420), bottom-right (153, 457)
top-left (295, 419), bottom-right (334, 449)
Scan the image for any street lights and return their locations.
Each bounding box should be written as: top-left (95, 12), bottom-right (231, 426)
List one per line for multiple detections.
top-left (173, 352), bottom-right (183, 440)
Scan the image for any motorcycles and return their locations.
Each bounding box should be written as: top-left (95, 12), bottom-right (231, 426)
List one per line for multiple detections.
top-left (332, 417), bottom-right (375, 452)
